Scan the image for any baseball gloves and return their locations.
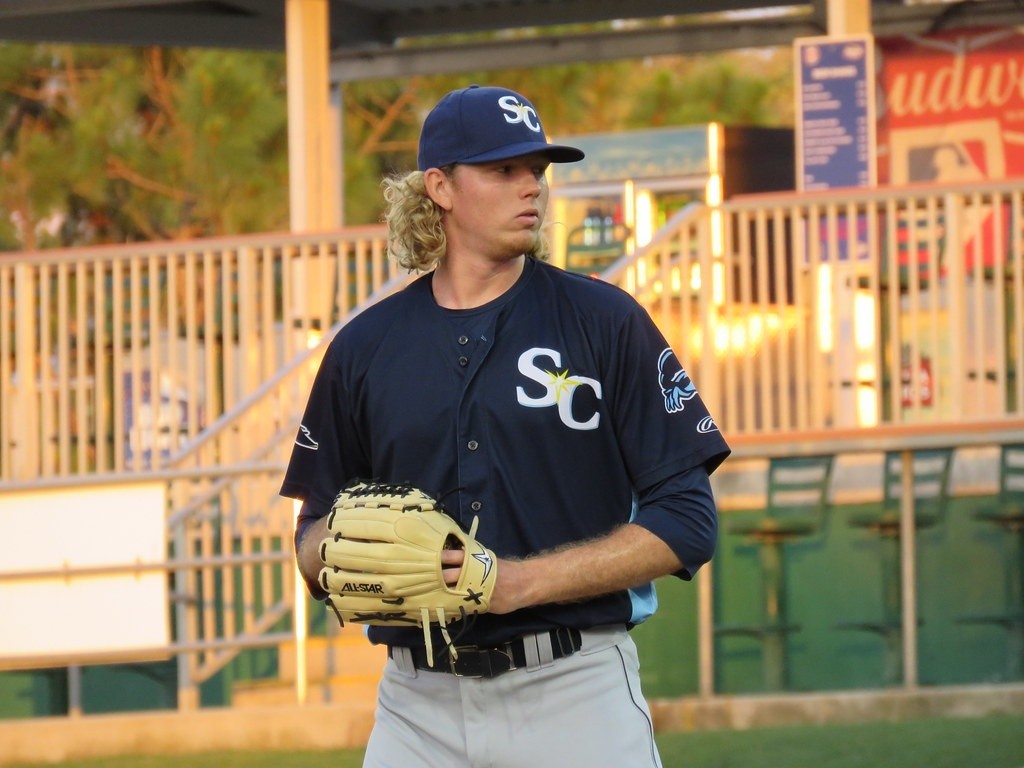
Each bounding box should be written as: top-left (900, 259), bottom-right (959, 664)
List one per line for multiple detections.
top-left (313, 473), bottom-right (498, 667)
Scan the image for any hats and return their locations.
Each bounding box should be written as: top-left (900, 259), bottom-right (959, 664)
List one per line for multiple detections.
top-left (418, 84), bottom-right (587, 174)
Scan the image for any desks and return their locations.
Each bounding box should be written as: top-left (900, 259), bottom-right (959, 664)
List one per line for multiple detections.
top-left (690, 430), bottom-right (1024, 705)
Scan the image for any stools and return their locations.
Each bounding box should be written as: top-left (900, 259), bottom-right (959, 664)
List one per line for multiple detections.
top-left (846, 446), bottom-right (953, 684)
top-left (957, 442), bottom-right (1024, 680)
top-left (715, 453), bottom-right (834, 691)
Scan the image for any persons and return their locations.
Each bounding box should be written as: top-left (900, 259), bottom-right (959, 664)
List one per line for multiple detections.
top-left (278, 84), bottom-right (732, 768)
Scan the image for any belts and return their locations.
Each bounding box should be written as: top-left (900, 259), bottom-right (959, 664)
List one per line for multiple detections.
top-left (392, 626), bottom-right (582, 677)
